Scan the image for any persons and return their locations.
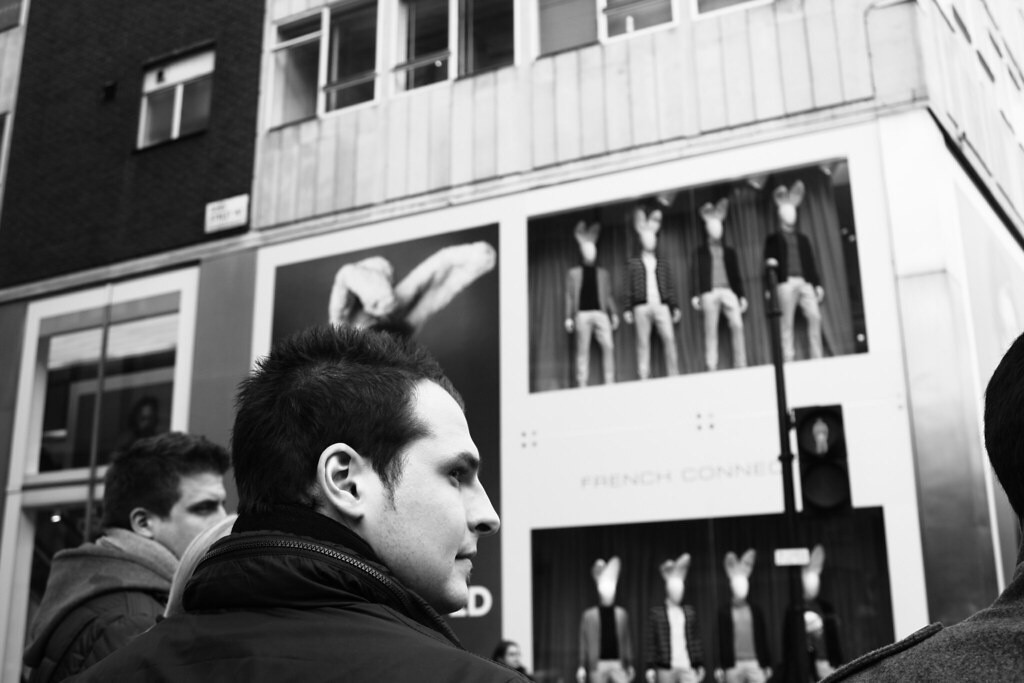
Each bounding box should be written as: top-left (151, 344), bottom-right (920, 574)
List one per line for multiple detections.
top-left (21, 430), bottom-right (236, 682)
top-left (818, 331), bottom-right (1024, 683)
top-left (711, 549), bottom-right (773, 683)
top-left (492, 640), bottom-right (528, 676)
top-left (620, 207), bottom-right (684, 376)
top-left (759, 180), bottom-right (825, 363)
top-left (563, 219), bottom-right (621, 387)
top-left (69, 326), bottom-right (537, 683)
top-left (781, 544), bottom-right (843, 683)
top-left (639, 552), bottom-right (708, 683)
top-left (575, 554), bottom-right (636, 683)
top-left (689, 197), bottom-right (750, 369)
